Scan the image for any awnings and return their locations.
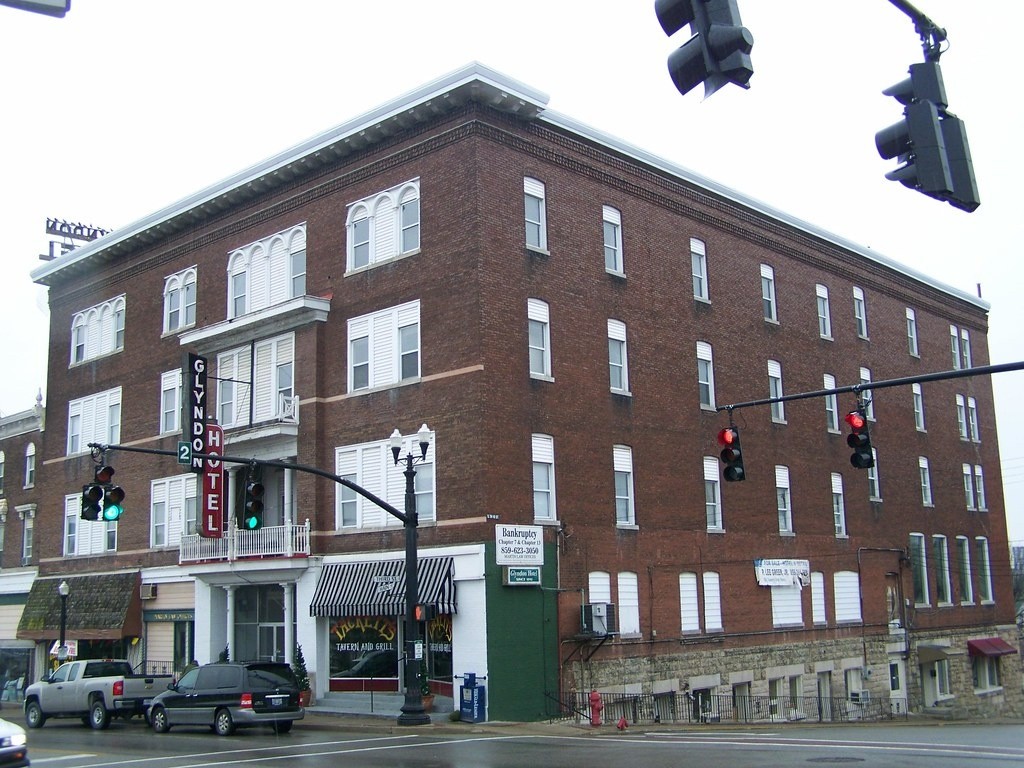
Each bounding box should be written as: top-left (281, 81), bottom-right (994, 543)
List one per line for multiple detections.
top-left (968, 637), bottom-right (1017, 658)
top-left (16, 571), bottom-right (141, 640)
top-left (917, 644), bottom-right (965, 664)
top-left (308, 557), bottom-right (458, 617)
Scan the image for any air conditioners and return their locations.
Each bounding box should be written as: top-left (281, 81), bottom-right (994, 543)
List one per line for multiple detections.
top-left (22, 556), bottom-right (32, 566)
top-left (850, 689), bottom-right (870, 704)
top-left (580, 603), bottom-right (616, 633)
top-left (140, 585), bottom-right (157, 600)
top-left (706, 713), bottom-right (719, 722)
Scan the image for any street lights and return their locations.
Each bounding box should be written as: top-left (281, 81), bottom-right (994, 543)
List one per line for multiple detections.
top-left (58, 581), bottom-right (69, 666)
top-left (390, 424), bottom-right (431, 727)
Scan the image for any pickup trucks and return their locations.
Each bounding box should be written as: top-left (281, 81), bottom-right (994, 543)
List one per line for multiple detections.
top-left (23, 659), bottom-right (176, 730)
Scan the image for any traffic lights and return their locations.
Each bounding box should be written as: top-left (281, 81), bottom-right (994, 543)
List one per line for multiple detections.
top-left (81, 483), bottom-right (103, 521)
top-left (655, 0), bottom-right (754, 97)
top-left (875, 62), bottom-right (982, 213)
top-left (94, 465), bottom-right (115, 485)
top-left (845, 410), bottom-right (875, 469)
top-left (243, 480), bottom-right (264, 530)
top-left (102, 485), bottom-right (125, 521)
top-left (414, 605), bottom-right (432, 621)
top-left (717, 426), bottom-right (746, 482)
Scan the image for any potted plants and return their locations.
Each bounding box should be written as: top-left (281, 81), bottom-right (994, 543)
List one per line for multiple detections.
top-left (421, 650), bottom-right (435, 711)
top-left (291, 640), bottom-right (312, 707)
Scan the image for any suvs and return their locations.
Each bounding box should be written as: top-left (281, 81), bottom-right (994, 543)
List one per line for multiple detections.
top-left (147, 659), bottom-right (305, 737)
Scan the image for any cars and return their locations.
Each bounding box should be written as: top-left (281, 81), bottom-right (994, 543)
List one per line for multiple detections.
top-left (0, 717), bottom-right (30, 768)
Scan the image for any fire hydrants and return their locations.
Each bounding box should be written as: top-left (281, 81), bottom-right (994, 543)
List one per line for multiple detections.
top-left (589, 689), bottom-right (603, 727)
